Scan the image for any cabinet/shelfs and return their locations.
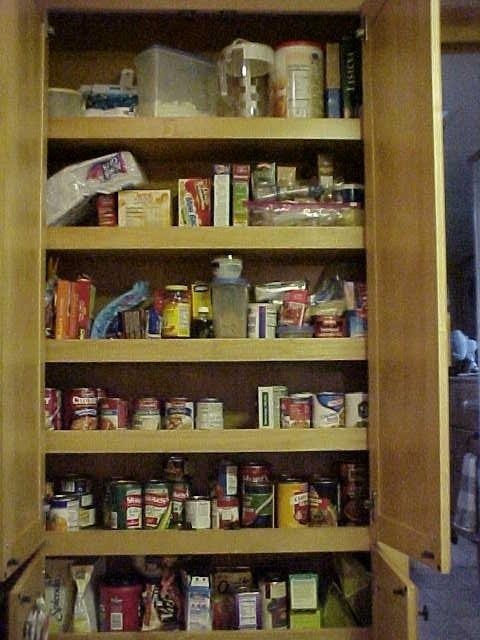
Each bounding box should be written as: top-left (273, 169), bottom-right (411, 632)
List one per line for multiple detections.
top-left (44, 1), bottom-right (373, 640)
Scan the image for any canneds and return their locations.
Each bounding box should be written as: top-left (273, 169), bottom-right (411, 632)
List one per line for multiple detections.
top-left (44, 455), bottom-right (367, 531)
top-left (161, 285), bottom-right (191, 338)
top-left (44, 388), bottom-right (368, 430)
